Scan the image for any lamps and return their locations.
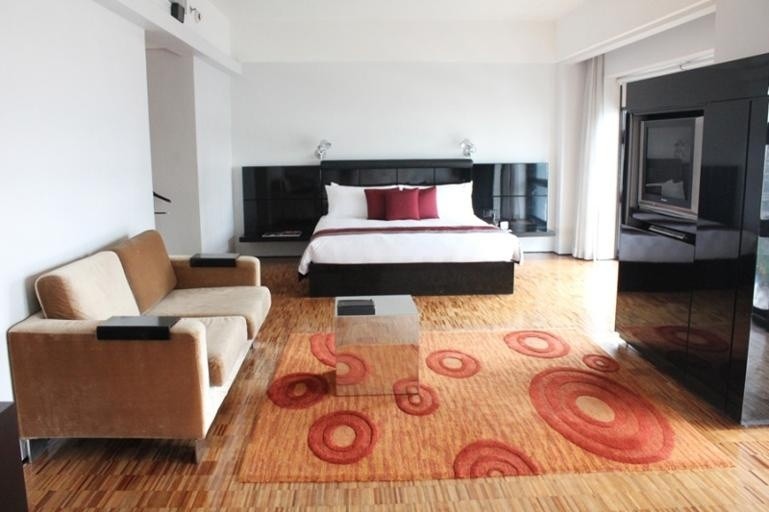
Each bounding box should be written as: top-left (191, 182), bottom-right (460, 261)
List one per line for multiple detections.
top-left (313, 138), bottom-right (332, 160)
top-left (458, 137), bottom-right (477, 158)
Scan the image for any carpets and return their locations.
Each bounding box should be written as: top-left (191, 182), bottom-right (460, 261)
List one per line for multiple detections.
top-left (239, 332), bottom-right (734, 483)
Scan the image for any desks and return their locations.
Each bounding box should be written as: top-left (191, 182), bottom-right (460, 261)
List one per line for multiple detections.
top-left (331, 294), bottom-right (422, 395)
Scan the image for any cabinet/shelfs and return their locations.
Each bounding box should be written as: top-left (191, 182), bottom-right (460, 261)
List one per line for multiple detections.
top-left (0, 401), bottom-right (31, 511)
top-left (612, 51), bottom-right (768, 428)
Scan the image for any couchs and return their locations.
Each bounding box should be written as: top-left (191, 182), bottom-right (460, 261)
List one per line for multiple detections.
top-left (6, 227), bottom-right (274, 464)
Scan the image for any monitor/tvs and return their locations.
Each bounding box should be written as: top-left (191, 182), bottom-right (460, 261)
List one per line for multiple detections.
top-left (638, 117), bottom-right (704, 220)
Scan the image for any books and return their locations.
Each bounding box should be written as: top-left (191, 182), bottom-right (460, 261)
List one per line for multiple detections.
top-left (337, 300), bottom-right (375, 316)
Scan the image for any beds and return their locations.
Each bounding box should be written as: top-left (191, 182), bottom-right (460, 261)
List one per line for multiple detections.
top-left (298, 158), bottom-right (524, 295)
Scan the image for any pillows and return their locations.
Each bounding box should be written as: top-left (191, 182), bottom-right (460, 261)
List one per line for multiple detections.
top-left (384, 188), bottom-right (421, 219)
top-left (398, 179), bottom-right (475, 214)
top-left (404, 185), bottom-right (439, 219)
top-left (329, 180), bottom-right (400, 219)
top-left (364, 188), bottom-right (399, 220)
top-left (325, 184), bottom-right (332, 215)
